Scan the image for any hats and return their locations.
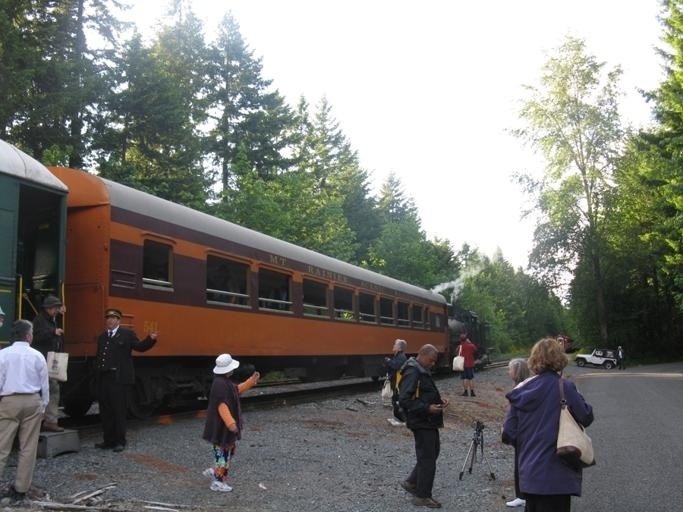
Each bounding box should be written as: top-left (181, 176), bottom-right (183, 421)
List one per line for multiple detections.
top-left (38, 294), bottom-right (63, 309)
top-left (212, 353), bottom-right (241, 375)
top-left (103, 309), bottom-right (123, 320)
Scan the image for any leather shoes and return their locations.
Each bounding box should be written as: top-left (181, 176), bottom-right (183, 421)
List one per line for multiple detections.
top-left (41, 421), bottom-right (64, 432)
top-left (113, 440), bottom-right (127, 453)
top-left (94, 441), bottom-right (117, 450)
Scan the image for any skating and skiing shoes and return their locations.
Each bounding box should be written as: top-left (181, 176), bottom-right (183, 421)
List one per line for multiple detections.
top-left (201, 467), bottom-right (214, 479)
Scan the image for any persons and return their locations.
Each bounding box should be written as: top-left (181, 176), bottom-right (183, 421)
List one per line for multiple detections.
top-left (95, 309), bottom-right (158, 452)
top-left (30, 295), bottom-right (67, 431)
top-left (615, 345), bottom-right (626, 370)
top-left (198, 352), bottom-right (261, 492)
top-left (380, 331), bottom-right (597, 512)
top-left (0, 318), bottom-right (49, 500)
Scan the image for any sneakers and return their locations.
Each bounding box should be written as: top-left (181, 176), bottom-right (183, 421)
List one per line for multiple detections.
top-left (413, 494), bottom-right (440, 508)
top-left (209, 480), bottom-right (234, 493)
top-left (400, 479), bottom-right (417, 495)
top-left (504, 497), bottom-right (527, 508)
top-left (7, 482), bottom-right (25, 498)
top-left (461, 390), bottom-right (476, 398)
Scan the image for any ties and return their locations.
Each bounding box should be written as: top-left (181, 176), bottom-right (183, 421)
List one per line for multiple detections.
top-left (108, 330), bottom-right (114, 339)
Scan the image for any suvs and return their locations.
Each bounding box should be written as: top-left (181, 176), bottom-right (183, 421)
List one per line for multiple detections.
top-left (572, 347), bottom-right (618, 370)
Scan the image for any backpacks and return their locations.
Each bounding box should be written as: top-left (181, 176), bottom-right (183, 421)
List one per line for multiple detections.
top-left (391, 362), bottom-right (422, 421)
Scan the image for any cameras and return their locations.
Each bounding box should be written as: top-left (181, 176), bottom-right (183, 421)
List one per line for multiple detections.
top-left (475, 421), bottom-right (484, 431)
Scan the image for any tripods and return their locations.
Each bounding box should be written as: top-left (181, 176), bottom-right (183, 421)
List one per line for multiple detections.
top-left (459, 430), bottom-right (496, 481)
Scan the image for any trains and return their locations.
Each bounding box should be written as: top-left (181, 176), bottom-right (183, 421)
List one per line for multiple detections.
top-left (0, 135), bottom-right (492, 419)
top-left (553, 335), bottom-right (573, 353)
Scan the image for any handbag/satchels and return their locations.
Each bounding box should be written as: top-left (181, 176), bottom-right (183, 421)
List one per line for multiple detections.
top-left (452, 344), bottom-right (468, 372)
top-left (45, 334), bottom-right (69, 382)
top-left (556, 377), bottom-right (596, 469)
